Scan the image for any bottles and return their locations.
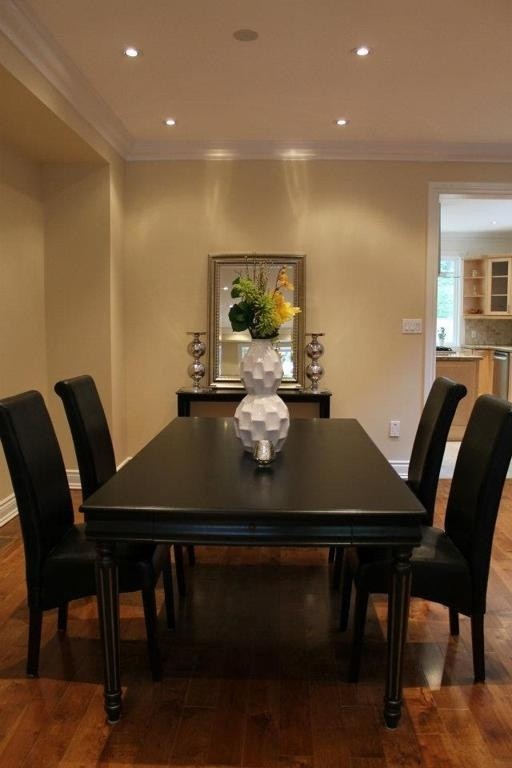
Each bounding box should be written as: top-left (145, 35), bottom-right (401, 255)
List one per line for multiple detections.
top-left (472, 283), bottom-right (477, 296)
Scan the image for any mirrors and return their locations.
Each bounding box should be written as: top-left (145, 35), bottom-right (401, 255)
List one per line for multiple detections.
top-left (203, 250), bottom-right (307, 391)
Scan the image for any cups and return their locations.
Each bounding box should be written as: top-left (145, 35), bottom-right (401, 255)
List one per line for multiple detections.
top-left (472, 269), bottom-right (477, 277)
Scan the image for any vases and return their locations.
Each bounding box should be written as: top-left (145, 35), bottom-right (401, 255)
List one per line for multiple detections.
top-left (186, 330), bottom-right (208, 391)
top-left (233, 338), bottom-right (290, 454)
top-left (303, 333), bottom-right (325, 393)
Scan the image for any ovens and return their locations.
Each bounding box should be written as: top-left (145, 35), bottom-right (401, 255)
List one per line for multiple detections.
top-left (493, 350), bottom-right (509, 400)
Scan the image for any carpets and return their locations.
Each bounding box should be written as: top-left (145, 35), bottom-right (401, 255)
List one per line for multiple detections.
top-left (439, 441), bottom-right (512, 480)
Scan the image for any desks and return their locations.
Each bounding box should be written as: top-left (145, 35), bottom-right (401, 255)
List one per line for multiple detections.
top-left (176, 386), bottom-right (332, 419)
top-left (79, 417), bottom-right (426, 728)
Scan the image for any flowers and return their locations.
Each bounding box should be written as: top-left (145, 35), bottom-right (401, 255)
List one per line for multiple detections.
top-left (229, 252), bottom-right (303, 338)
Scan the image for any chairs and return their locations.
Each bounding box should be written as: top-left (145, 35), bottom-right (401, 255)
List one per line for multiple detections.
top-left (327, 375), bottom-right (468, 593)
top-left (0, 390), bottom-right (176, 681)
top-left (55, 374), bottom-right (196, 611)
top-left (340, 395), bottom-right (512, 683)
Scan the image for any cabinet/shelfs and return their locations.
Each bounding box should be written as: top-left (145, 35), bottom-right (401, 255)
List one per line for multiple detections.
top-left (436, 361), bottom-right (479, 442)
top-left (463, 253), bottom-right (512, 320)
